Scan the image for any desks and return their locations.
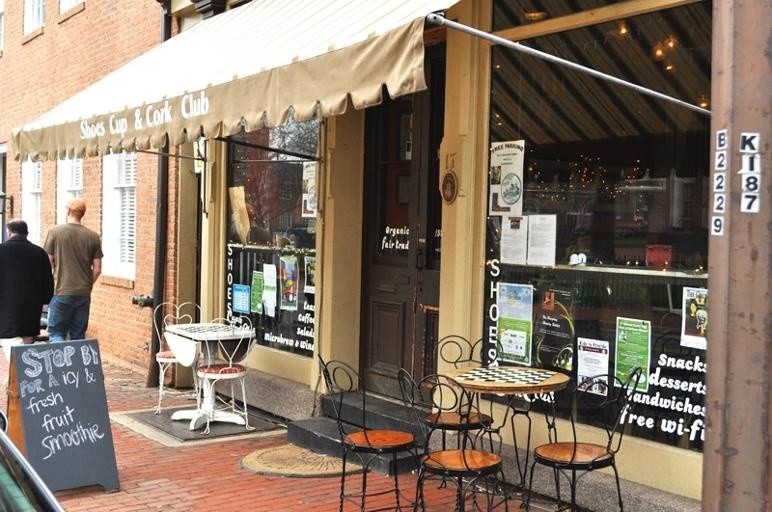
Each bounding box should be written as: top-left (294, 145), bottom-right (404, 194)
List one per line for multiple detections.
top-left (453, 365), bottom-right (572, 511)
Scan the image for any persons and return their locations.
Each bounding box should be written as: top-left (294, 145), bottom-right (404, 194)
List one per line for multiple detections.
top-left (686, 299), bottom-right (699, 336)
top-left (0, 197), bottom-right (105, 365)
top-left (558, 231), bottom-right (609, 339)
top-left (228, 203), bottom-right (275, 287)
top-left (283, 207), bottom-right (314, 253)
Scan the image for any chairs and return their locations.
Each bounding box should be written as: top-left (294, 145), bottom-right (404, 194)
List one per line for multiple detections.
top-left (318, 353), bottom-right (427, 512)
top-left (524, 363), bottom-right (643, 511)
top-left (395, 366), bottom-right (510, 512)
top-left (152, 303), bottom-right (255, 436)
top-left (423, 333), bottom-right (508, 490)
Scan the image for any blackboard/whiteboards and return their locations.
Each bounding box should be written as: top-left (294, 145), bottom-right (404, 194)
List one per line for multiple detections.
top-left (11, 338), bottom-right (118, 490)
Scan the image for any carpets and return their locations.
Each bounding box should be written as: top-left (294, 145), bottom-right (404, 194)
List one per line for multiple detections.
top-left (243, 441), bottom-right (368, 476)
